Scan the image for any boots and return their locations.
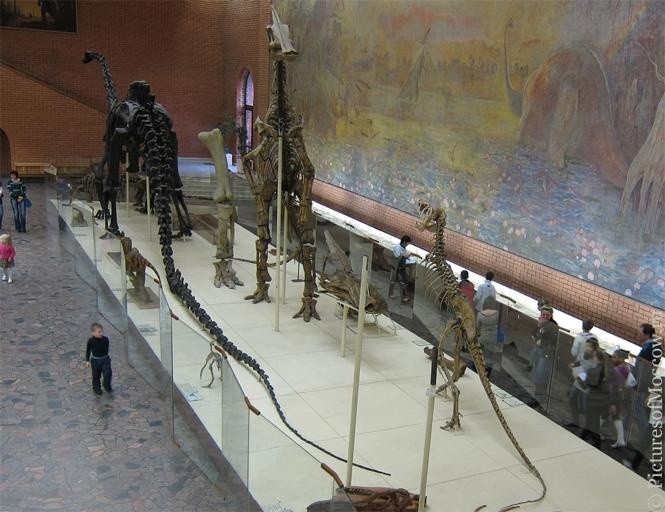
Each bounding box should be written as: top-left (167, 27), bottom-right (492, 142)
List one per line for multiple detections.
top-left (611, 419), bottom-right (627, 448)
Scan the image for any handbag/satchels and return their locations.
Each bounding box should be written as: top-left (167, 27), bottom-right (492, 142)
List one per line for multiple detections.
top-left (497, 329), bottom-right (504, 343)
top-left (24, 198), bottom-right (32, 208)
top-left (624, 373), bottom-right (637, 388)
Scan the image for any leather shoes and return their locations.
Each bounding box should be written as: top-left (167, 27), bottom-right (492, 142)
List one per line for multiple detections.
top-left (564, 423), bottom-right (578, 427)
top-left (527, 398), bottom-right (539, 407)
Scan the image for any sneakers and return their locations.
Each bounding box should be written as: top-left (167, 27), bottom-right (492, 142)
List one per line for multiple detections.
top-left (94, 385), bottom-right (112, 394)
top-left (2, 276), bottom-right (12, 283)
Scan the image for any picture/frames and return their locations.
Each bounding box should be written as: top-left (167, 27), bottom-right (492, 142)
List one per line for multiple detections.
top-left (0, 0), bottom-right (79, 34)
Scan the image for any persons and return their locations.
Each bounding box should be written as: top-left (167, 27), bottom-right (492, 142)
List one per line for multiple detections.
top-left (530, 298), bottom-right (559, 408)
top-left (0, 234), bottom-right (16, 284)
top-left (387, 235), bottom-right (422, 303)
top-left (458, 270), bottom-right (499, 380)
top-left (562, 318), bottom-right (665, 486)
top-left (0, 171), bottom-right (27, 233)
top-left (85, 323), bottom-right (114, 396)
